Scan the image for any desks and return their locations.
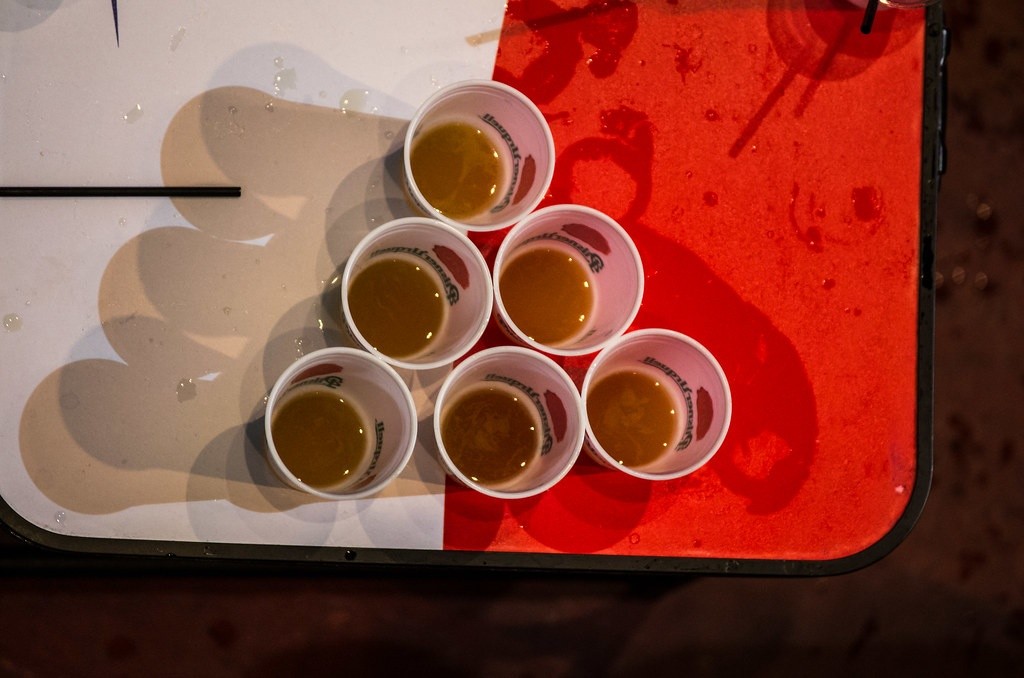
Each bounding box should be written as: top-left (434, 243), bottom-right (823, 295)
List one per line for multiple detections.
top-left (1, 0), bottom-right (950, 580)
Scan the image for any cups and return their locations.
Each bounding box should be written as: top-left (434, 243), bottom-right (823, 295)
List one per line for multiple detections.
top-left (402, 80), bottom-right (554, 234)
top-left (433, 346), bottom-right (585, 500)
top-left (580, 329), bottom-right (732, 481)
top-left (494, 204), bottom-right (644, 360)
top-left (265, 348), bottom-right (419, 501)
top-left (339, 217), bottom-right (493, 373)
